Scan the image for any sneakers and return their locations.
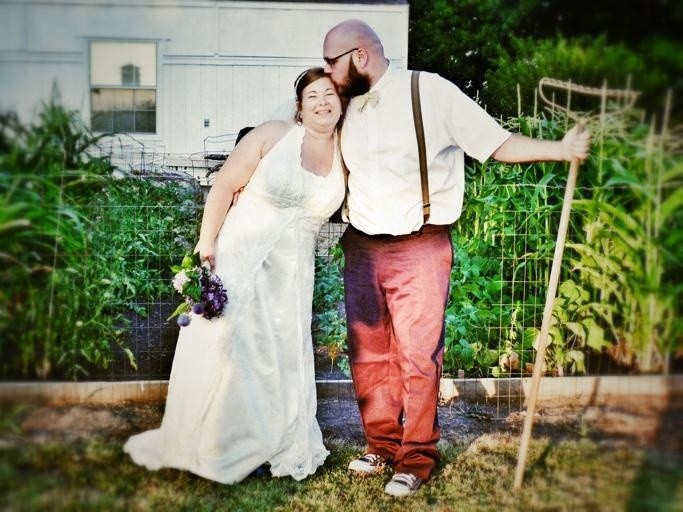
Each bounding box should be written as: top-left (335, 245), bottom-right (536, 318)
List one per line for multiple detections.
top-left (349, 453), bottom-right (385, 473)
top-left (385, 474), bottom-right (422, 497)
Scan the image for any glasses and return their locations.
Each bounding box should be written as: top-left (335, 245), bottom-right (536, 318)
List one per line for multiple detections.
top-left (323, 47), bottom-right (360, 66)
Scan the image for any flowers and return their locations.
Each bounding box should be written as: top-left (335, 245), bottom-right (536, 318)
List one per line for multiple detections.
top-left (164, 254), bottom-right (228, 328)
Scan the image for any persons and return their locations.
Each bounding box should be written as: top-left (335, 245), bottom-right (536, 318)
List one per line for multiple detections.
top-left (231, 21), bottom-right (592, 498)
top-left (120, 67), bottom-right (344, 489)
